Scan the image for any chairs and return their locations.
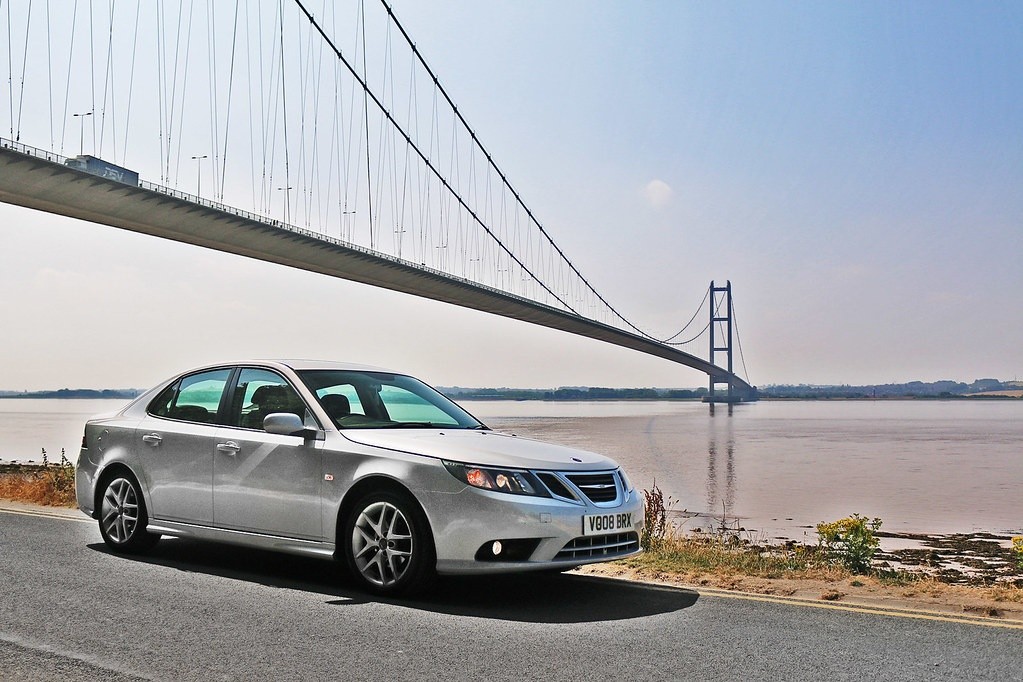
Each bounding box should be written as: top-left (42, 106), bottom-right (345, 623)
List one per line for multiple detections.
top-left (174, 406), bottom-right (212, 423)
top-left (322, 394), bottom-right (351, 418)
top-left (285, 385), bottom-right (306, 425)
top-left (243, 385), bottom-right (289, 430)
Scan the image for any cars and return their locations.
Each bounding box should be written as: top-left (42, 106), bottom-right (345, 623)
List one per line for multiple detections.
top-left (75, 358), bottom-right (646, 598)
